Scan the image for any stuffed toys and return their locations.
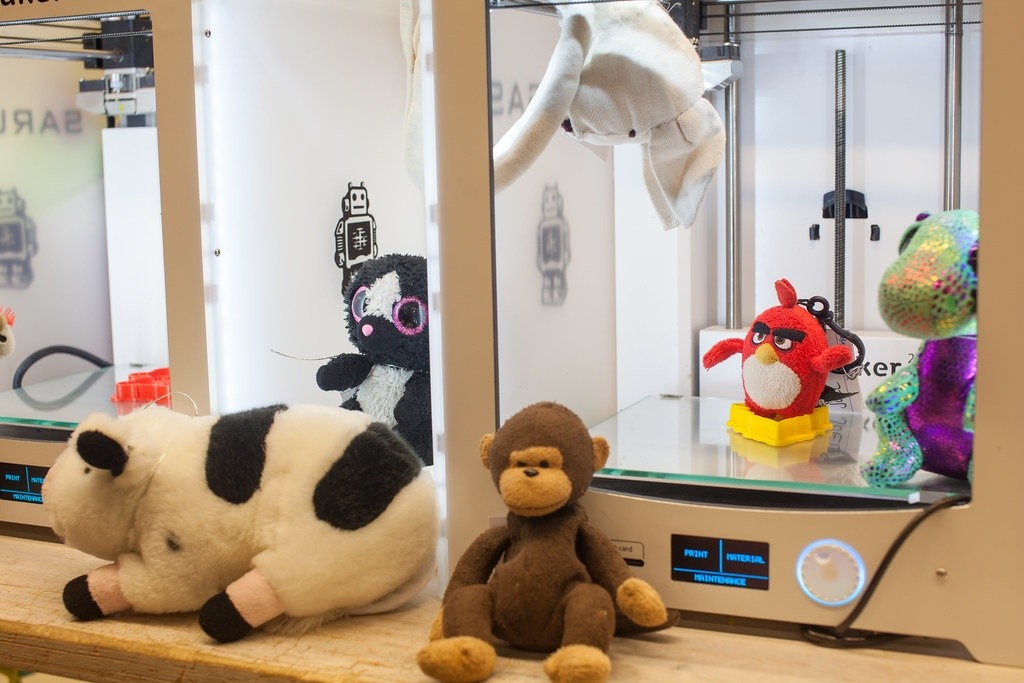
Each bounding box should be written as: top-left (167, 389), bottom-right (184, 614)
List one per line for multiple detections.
top-left (40, 255), bottom-right (852, 682)
